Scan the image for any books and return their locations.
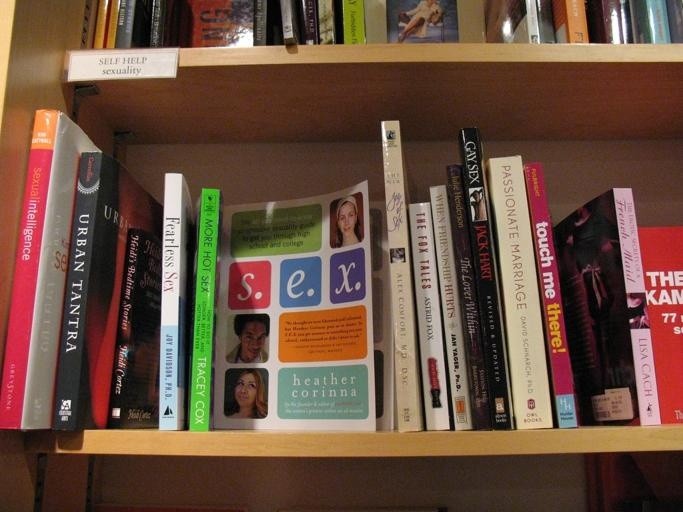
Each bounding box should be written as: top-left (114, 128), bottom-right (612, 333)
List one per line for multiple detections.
top-left (2, 109), bottom-right (683, 433)
top-left (484, 1), bottom-right (683, 44)
top-left (81, 0), bottom-right (365, 49)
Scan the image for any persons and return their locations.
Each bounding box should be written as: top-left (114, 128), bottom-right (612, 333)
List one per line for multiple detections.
top-left (398, 0), bottom-right (442, 41)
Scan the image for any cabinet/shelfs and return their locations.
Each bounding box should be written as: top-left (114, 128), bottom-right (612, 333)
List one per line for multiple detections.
top-left (0, 0), bottom-right (683, 512)
